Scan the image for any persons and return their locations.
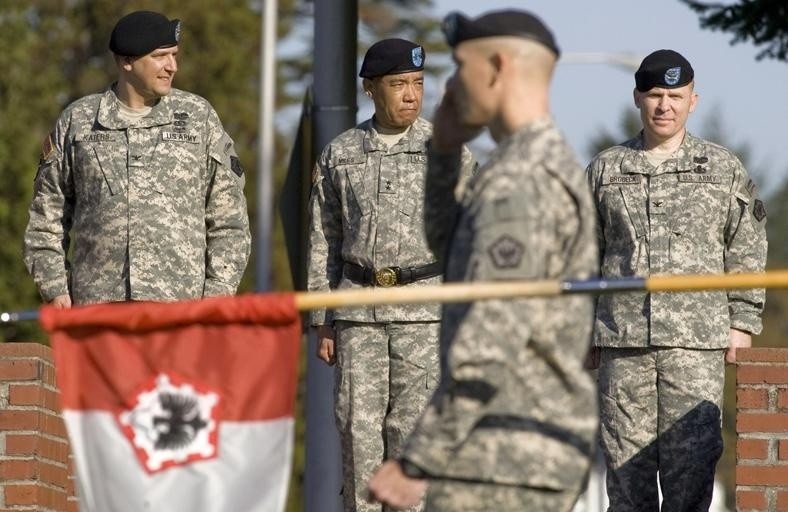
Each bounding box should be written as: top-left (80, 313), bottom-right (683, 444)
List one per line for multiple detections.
top-left (365, 11), bottom-right (600, 512)
top-left (584, 50), bottom-right (768, 512)
top-left (306, 38), bottom-right (479, 512)
top-left (22, 12), bottom-right (251, 311)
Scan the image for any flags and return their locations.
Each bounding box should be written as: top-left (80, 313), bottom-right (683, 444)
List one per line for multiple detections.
top-left (40, 292), bottom-right (299, 512)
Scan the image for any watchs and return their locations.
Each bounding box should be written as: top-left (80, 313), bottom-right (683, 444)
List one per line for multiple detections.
top-left (401, 457), bottom-right (428, 479)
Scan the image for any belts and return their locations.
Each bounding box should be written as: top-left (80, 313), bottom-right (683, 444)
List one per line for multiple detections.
top-left (342, 261), bottom-right (447, 286)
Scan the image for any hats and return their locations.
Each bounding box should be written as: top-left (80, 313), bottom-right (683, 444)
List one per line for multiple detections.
top-left (110, 11), bottom-right (183, 56)
top-left (635, 49), bottom-right (695, 92)
top-left (359, 38), bottom-right (427, 79)
top-left (441, 9), bottom-right (563, 54)
